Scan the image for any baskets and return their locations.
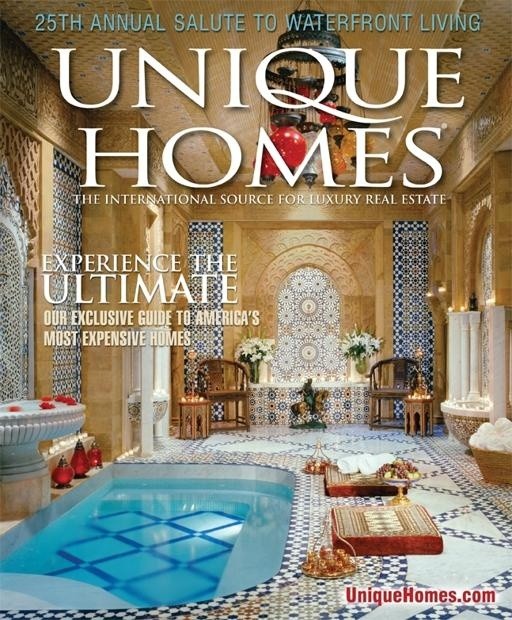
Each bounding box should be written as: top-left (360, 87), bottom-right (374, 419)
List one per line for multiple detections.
top-left (469, 446), bottom-right (512, 486)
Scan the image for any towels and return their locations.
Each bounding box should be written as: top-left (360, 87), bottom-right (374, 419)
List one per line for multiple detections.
top-left (336, 452), bottom-right (394, 476)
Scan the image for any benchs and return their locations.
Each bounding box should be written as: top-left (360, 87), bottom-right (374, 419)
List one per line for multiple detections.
top-left (330, 504), bottom-right (444, 558)
top-left (322, 464), bottom-right (407, 497)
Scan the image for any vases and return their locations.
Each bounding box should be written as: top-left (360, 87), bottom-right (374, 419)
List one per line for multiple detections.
top-left (249, 361), bottom-right (259, 383)
top-left (355, 357), bottom-right (368, 384)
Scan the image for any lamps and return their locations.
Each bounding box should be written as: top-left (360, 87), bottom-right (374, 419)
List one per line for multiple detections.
top-left (249, 1), bottom-right (374, 192)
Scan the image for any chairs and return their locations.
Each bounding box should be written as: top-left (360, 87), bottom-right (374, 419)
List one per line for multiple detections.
top-left (366, 356), bottom-right (421, 431)
top-left (196, 358), bottom-right (252, 436)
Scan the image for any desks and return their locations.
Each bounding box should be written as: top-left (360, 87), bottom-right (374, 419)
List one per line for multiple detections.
top-left (401, 398), bottom-right (434, 438)
top-left (178, 400), bottom-right (211, 440)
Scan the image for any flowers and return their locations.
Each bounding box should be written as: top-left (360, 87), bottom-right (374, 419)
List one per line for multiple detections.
top-left (337, 321), bottom-right (386, 365)
top-left (233, 325), bottom-right (277, 367)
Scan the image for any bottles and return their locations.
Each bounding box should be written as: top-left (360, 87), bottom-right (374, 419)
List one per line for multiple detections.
top-left (87, 441), bottom-right (103, 471)
top-left (51, 454), bottom-right (76, 490)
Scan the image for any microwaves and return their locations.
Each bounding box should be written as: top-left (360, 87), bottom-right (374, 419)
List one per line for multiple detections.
top-left (70, 439), bottom-right (90, 480)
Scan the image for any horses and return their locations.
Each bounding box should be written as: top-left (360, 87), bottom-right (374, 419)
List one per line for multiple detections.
top-left (290, 389), bottom-right (330, 428)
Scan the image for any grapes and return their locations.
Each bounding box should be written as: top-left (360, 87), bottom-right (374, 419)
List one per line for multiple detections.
top-left (376, 461), bottom-right (416, 489)
